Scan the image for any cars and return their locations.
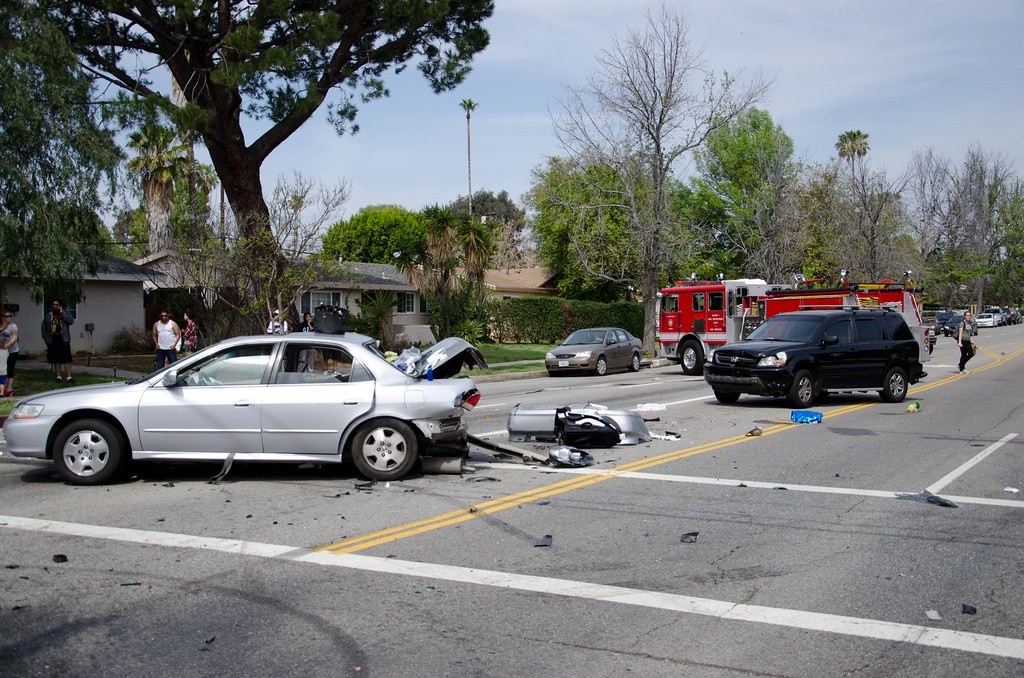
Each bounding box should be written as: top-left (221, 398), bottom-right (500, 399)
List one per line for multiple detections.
top-left (926, 308), bottom-right (1022, 337)
top-left (544, 327), bottom-right (644, 376)
top-left (1, 332), bottom-right (488, 487)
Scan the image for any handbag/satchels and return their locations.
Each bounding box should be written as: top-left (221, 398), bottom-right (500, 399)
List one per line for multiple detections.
top-left (791, 410), bottom-right (823, 424)
top-left (315, 305), bottom-right (349, 334)
top-left (971, 343), bottom-right (977, 356)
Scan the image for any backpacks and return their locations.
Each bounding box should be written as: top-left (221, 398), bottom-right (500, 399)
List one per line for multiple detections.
top-left (953, 320), bottom-right (966, 339)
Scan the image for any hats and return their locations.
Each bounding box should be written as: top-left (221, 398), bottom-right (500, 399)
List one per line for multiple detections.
top-left (273, 309), bottom-right (279, 315)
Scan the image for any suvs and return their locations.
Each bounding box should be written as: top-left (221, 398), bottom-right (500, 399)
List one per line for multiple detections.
top-left (702, 304), bottom-right (931, 408)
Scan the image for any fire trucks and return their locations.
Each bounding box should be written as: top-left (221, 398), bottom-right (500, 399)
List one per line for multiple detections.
top-left (655, 272), bottom-right (939, 376)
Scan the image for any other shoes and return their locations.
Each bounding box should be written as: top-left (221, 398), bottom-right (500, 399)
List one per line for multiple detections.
top-left (956, 363), bottom-right (961, 372)
top-left (55, 378), bottom-right (61, 383)
top-left (4, 390), bottom-right (14, 396)
top-left (66, 378), bottom-right (73, 382)
top-left (323, 371), bottom-right (328, 376)
top-left (960, 369), bottom-right (970, 375)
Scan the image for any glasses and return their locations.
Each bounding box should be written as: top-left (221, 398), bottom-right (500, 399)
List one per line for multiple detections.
top-left (4, 315), bottom-right (13, 318)
top-left (160, 314), bottom-right (168, 318)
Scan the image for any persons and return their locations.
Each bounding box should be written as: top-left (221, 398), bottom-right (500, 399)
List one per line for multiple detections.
top-left (153, 310), bottom-right (182, 370)
top-left (958, 311), bottom-right (974, 375)
top-left (298, 313), bottom-right (316, 378)
top-left (0, 310), bottom-right (20, 397)
top-left (183, 309), bottom-right (197, 356)
top-left (41, 300), bottom-right (76, 383)
top-left (267, 309), bottom-right (288, 335)
top-left (322, 349), bottom-right (342, 376)
